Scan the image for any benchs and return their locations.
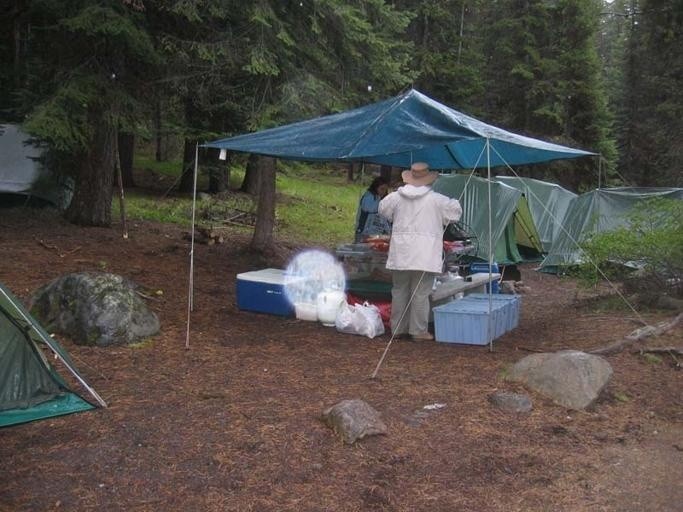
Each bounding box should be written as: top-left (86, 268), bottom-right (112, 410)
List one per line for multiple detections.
top-left (429, 272), bottom-right (501, 307)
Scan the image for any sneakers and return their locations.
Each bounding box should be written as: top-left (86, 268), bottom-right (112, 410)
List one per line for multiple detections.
top-left (410, 333), bottom-right (433, 342)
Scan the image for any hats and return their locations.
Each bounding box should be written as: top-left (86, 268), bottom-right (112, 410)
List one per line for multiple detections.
top-left (401, 162), bottom-right (438, 185)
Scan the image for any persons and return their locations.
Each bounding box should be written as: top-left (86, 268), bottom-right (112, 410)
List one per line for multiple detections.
top-left (379, 162), bottom-right (463, 339)
top-left (355, 178), bottom-right (389, 242)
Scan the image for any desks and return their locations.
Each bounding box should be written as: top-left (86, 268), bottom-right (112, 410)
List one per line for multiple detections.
top-left (335, 240), bottom-right (475, 260)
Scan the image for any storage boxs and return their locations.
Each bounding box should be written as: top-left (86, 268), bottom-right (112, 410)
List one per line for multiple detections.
top-left (432, 292), bottom-right (522, 346)
top-left (236, 267), bottom-right (294, 316)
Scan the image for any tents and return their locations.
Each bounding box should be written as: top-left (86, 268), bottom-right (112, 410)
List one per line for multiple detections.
top-left (0, 284), bottom-right (107, 430)
top-left (535, 185), bottom-right (683, 273)
top-left (434, 172), bottom-right (546, 269)
top-left (492, 175), bottom-right (577, 254)
